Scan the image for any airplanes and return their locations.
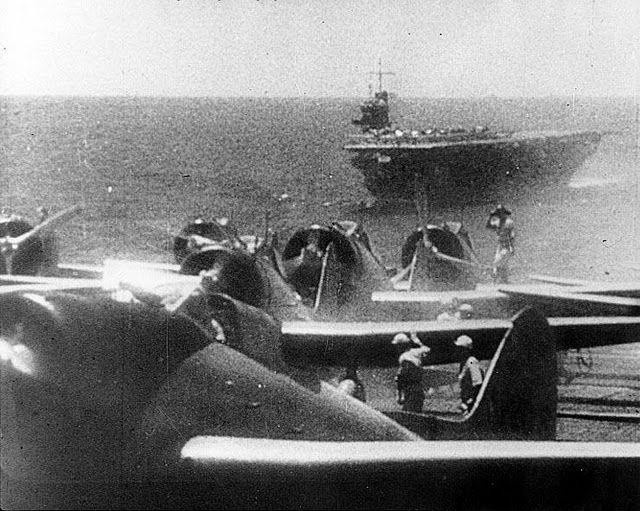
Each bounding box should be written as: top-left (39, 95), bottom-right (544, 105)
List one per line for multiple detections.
top-left (0, 205), bottom-right (640, 511)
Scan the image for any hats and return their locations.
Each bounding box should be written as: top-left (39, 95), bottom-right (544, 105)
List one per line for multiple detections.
top-left (454, 336), bottom-right (474, 351)
top-left (490, 204), bottom-right (512, 218)
top-left (390, 333), bottom-right (410, 347)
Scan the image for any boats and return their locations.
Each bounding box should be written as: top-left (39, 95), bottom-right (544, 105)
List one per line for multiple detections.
top-left (346, 56), bottom-right (603, 194)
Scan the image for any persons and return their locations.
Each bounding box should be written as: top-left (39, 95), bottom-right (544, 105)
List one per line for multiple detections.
top-left (388, 332), bottom-right (432, 413)
top-left (338, 354), bottom-right (367, 403)
top-left (432, 299), bottom-right (477, 322)
top-left (485, 204), bottom-right (519, 286)
top-left (453, 332), bottom-right (485, 412)
